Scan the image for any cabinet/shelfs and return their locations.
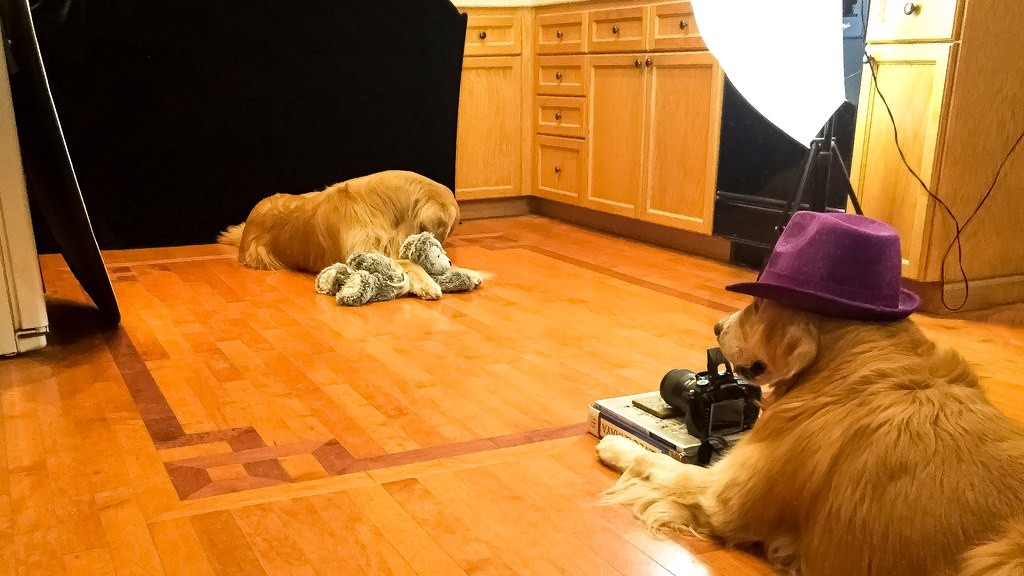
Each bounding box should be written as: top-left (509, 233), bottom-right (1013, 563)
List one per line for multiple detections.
top-left (531, 1), bottom-right (588, 214)
top-left (845, 0), bottom-right (1024, 284)
top-left (583, 0), bottom-right (726, 237)
top-left (455, 6), bottom-right (531, 202)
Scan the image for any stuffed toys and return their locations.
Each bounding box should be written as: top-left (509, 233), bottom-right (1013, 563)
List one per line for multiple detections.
top-left (313, 231), bottom-right (482, 308)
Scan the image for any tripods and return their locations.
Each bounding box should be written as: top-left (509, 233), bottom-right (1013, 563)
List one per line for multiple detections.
top-left (780, 111), bottom-right (864, 237)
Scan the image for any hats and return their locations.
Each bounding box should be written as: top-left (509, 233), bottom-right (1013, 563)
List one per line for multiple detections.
top-left (725, 210), bottom-right (923, 323)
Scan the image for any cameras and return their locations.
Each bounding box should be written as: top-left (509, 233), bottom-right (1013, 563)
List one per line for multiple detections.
top-left (660, 347), bottom-right (769, 437)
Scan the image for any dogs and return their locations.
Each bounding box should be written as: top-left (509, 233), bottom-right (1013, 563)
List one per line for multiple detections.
top-left (593, 293), bottom-right (1024, 576)
top-left (216, 169), bottom-right (496, 300)
